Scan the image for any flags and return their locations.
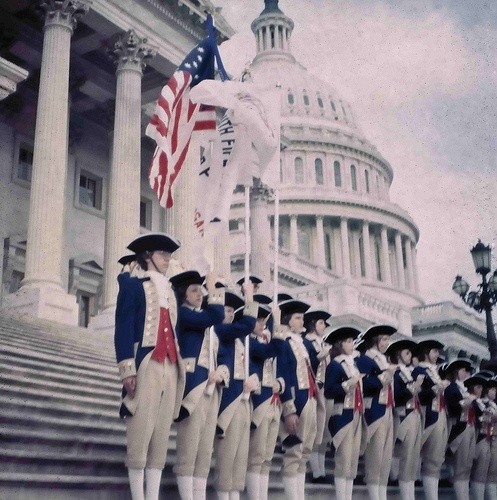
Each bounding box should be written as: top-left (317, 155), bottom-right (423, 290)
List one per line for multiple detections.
top-left (145, 14), bottom-right (280, 276)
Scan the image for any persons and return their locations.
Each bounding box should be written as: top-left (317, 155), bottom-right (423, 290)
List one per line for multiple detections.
top-left (113, 234), bottom-right (497, 500)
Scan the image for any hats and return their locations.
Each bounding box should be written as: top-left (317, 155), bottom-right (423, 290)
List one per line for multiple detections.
top-left (303, 311), bottom-right (331, 320)
top-left (354, 334), bottom-right (365, 351)
top-left (118, 255), bottom-right (137, 266)
top-left (384, 340), bottom-right (418, 357)
top-left (168, 270), bottom-right (206, 285)
top-left (203, 281), bottom-right (225, 291)
top-left (235, 305), bottom-right (272, 319)
top-left (323, 327), bottom-right (361, 345)
top-left (438, 359), bottom-right (497, 394)
top-left (278, 293), bottom-right (293, 302)
top-left (279, 301), bottom-right (311, 313)
top-left (224, 292), bottom-right (245, 313)
top-left (361, 325), bottom-right (397, 342)
top-left (126, 234), bottom-right (181, 253)
top-left (236, 276), bottom-right (263, 286)
top-left (418, 339), bottom-right (445, 357)
top-left (253, 295), bottom-right (273, 304)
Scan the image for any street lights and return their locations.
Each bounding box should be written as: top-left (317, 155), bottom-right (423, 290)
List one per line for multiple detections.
top-left (451, 238), bottom-right (497, 366)
